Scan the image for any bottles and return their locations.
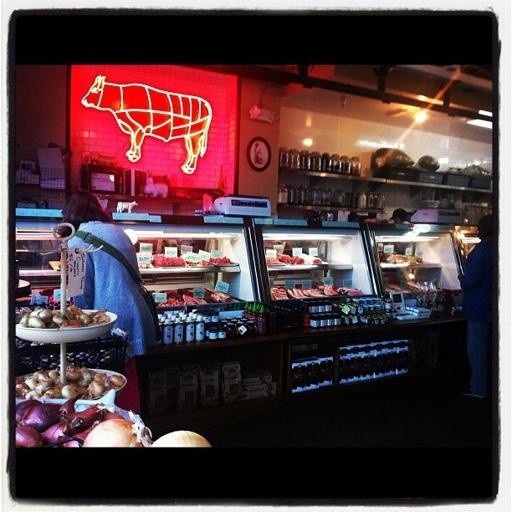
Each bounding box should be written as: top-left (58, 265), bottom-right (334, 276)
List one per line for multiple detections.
top-left (277, 183), bottom-right (385, 210)
top-left (244, 297), bottom-right (310, 336)
top-left (420, 281), bottom-right (437, 312)
top-left (280, 148), bottom-right (362, 176)
top-left (156, 310), bottom-right (205, 344)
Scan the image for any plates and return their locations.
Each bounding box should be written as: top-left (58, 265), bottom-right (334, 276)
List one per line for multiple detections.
top-left (16, 367), bottom-right (127, 407)
top-left (14, 308), bottom-right (118, 344)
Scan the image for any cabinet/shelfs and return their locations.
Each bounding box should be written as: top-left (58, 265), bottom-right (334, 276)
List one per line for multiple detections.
top-left (15, 167), bottom-right (498, 441)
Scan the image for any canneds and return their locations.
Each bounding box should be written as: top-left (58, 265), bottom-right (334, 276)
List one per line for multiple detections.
top-left (309, 297), bottom-right (392, 328)
top-left (199, 310), bottom-right (255, 342)
top-left (278, 146), bottom-right (386, 210)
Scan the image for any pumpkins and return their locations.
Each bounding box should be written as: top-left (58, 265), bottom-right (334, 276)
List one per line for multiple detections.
top-left (82, 419), bottom-right (144, 447)
top-left (149, 430), bottom-right (211, 447)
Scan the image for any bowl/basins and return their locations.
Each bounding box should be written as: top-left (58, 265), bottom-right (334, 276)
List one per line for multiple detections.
top-left (421, 200), bottom-right (439, 208)
top-left (49, 261), bottom-right (62, 271)
top-left (438, 201), bottom-right (449, 208)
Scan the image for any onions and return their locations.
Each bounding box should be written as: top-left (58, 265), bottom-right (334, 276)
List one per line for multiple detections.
top-left (16, 367), bottom-right (124, 399)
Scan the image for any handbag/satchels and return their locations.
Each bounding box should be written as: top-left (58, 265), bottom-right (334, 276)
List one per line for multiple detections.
top-left (142, 290), bottom-right (163, 343)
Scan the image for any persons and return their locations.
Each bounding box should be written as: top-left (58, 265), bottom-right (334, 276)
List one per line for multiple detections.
top-left (459, 214), bottom-right (495, 401)
top-left (391, 206), bottom-right (417, 224)
top-left (60, 189), bottom-right (163, 418)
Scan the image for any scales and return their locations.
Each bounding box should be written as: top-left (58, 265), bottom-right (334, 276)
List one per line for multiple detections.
top-left (410, 207), bottom-right (462, 225)
top-left (211, 194), bottom-right (272, 217)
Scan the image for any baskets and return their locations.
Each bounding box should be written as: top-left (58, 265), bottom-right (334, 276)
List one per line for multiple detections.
top-left (13, 327), bottom-right (129, 373)
top-left (373, 162), bottom-right (492, 189)
